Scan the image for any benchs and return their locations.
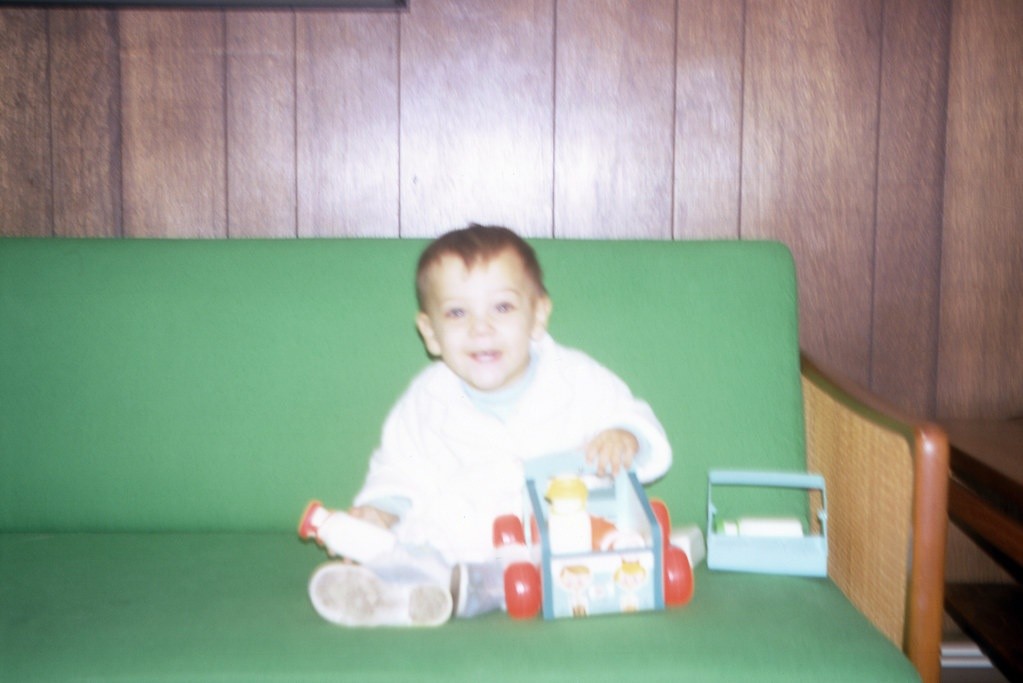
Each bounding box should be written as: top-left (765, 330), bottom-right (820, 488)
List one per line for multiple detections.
top-left (0, 236), bottom-right (948, 682)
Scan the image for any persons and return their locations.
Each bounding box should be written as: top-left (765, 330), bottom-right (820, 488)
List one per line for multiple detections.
top-left (306, 223), bottom-right (676, 628)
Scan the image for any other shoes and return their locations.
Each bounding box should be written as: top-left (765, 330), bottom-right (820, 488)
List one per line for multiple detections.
top-left (309, 561), bottom-right (454, 627)
